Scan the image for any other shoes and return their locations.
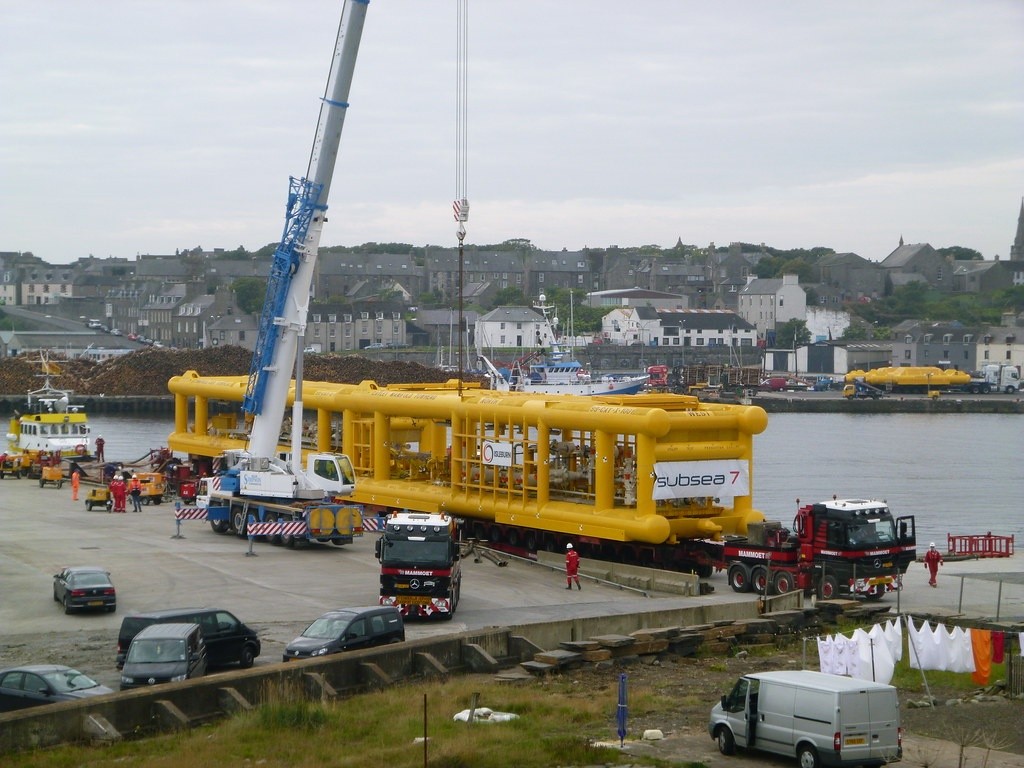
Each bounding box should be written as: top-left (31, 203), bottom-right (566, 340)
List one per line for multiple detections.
top-left (578, 585), bottom-right (581, 590)
top-left (113, 507), bottom-right (142, 513)
top-left (566, 587), bottom-right (571, 590)
top-left (933, 585), bottom-right (937, 588)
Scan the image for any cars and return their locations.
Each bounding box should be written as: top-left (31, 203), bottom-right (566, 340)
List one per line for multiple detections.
top-left (303, 346), bottom-right (317, 354)
top-left (387, 340), bottom-right (410, 349)
top-left (1, 665), bottom-right (117, 713)
top-left (364, 342), bottom-right (386, 350)
top-left (88, 319), bottom-right (180, 352)
top-left (53, 565), bottom-right (116, 615)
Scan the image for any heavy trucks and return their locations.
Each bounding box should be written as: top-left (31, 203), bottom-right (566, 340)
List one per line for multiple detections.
top-left (460, 491), bottom-right (918, 601)
top-left (644, 356), bottom-right (1022, 397)
top-left (195, 473), bottom-right (364, 549)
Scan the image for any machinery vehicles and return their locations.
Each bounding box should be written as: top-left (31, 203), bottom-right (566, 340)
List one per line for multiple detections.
top-left (195, 0), bottom-right (470, 508)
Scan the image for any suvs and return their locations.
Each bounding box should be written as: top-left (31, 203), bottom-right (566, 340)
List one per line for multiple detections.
top-left (282, 603), bottom-right (405, 692)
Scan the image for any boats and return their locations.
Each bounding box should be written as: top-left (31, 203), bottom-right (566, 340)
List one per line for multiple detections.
top-left (5, 345), bottom-right (92, 460)
top-left (478, 291), bottom-right (651, 397)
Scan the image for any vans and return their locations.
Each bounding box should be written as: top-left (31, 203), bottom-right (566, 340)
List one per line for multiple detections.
top-left (120, 622), bottom-right (210, 708)
top-left (117, 604), bottom-right (262, 676)
top-left (708, 669), bottom-right (903, 768)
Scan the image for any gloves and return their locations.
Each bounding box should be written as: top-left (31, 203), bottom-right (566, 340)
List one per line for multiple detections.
top-left (940, 560), bottom-right (943, 566)
top-left (924, 563), bottom-right (927, 569)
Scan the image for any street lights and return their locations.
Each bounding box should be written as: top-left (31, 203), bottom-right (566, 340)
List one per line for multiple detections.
top-left (923, 321), bottom-right (940, 367)
top-left (859, 320), bottom-right (879, 371)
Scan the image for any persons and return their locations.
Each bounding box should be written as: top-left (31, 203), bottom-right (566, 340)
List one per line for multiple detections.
top-left (924, 543), bottom-right (944, 588)
top-left (72, 469), bottom-right (80, 501)
top-left (109, 474), bottom-right (129, 513)
top-left (128, 474), bottom-right (142, 512)
top-left (565, 543), bottom-right (582, 590)
top-left (0, 449), bottom-right (61, 476)
top-left (95, 435), bottom-right (105, 464)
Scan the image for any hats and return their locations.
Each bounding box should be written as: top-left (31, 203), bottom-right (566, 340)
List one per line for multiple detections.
top-left (75, 469), bottom-right (80, 472)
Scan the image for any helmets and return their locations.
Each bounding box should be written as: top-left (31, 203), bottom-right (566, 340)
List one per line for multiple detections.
top-left (930, 543), bottom-right (935, 547)
top-left (4, 452), bottom-right (8, 456)
top-left (566, 543), bottom-right (573, 548)
top-left (98, 435), bottom-right (103, 440)
top-left (132, 475), bottom-right (137, 479)
top-left (118, 476), bottom-right (124, 481)
top-left (113, 476), bottom-right (119, 480)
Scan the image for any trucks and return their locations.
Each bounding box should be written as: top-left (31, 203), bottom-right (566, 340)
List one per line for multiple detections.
top-left (842, 384), bottom-right (884, 400)
top-left (375, 510), bottom-right (466, 622)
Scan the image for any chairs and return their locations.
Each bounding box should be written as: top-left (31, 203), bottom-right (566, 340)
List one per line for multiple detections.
top-left (372, 619), bottom-right (384, 632)
